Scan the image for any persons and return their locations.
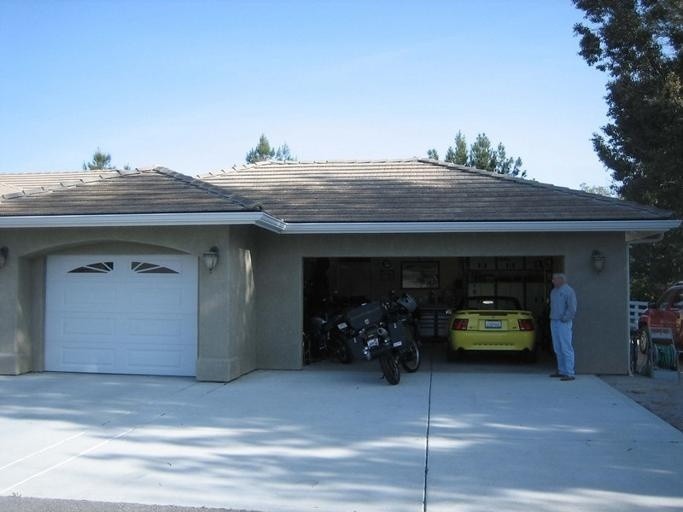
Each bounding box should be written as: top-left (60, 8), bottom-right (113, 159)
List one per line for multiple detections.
top-left (549, 273), bottom-right (578, 382)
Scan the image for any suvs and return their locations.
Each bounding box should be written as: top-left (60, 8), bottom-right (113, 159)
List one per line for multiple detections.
top-left (639, 287), bottom-right (683, 362)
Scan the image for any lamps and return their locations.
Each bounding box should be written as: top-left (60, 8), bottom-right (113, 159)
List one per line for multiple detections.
top-left (202, 247), bottom-right (219, 273)
top-left (590, 247), bottom-right (608, 275)
top-left (0, 246), bottom-right (10, 269)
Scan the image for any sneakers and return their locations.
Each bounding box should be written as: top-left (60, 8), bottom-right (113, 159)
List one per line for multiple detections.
top-left (549, 372), bottom-right (564, 377)
top-left (559, 375), bottom-right (576, 380)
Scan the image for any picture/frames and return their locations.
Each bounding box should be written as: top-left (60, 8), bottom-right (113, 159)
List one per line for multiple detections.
top-left (400, 260), bottom-right (440, 290)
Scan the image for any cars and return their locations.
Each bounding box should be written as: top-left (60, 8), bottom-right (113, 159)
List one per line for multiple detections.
top-left (444, 296), bottom-right (537, 363)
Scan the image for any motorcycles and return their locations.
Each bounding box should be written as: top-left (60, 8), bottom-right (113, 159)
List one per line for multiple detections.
top-left (308, 313), bottom-right (351, 363)
top-left (353, 292), bottom-right (421, 384)
